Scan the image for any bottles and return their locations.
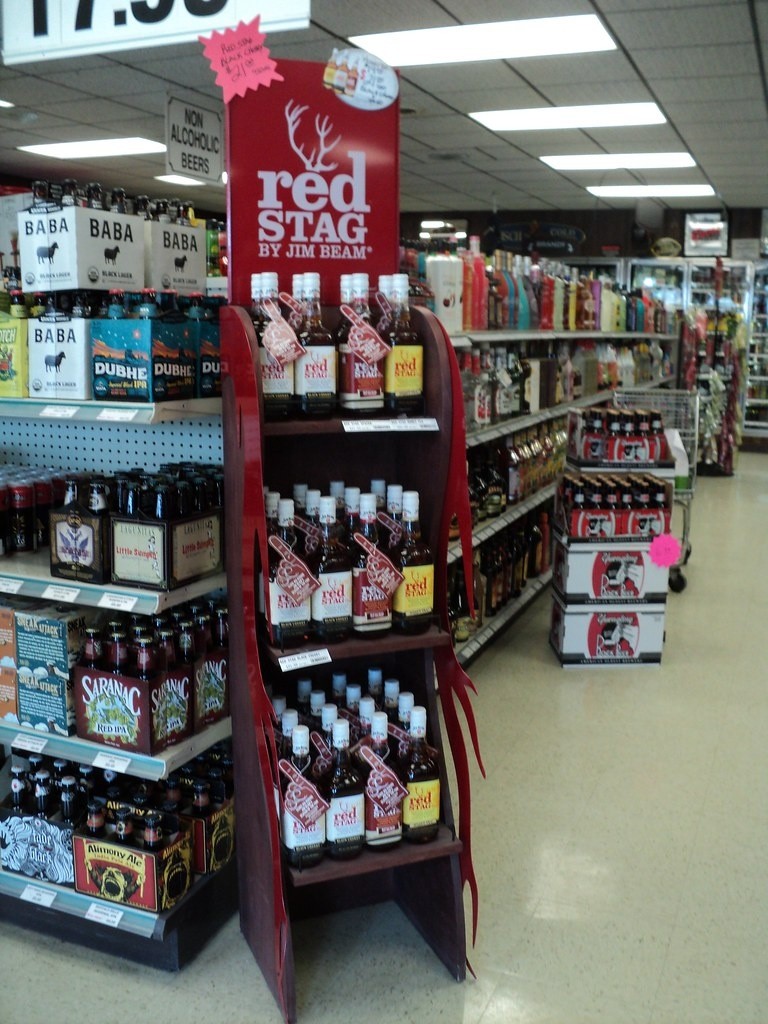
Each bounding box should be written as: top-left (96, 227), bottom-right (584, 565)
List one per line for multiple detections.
top-left (5, 741), bottom-right (232, 849)
top-left (266, 669), bottom-right (441, 869)
top-left (0, 178), bottom-right (228, 321)
top-left (85, 597), bottom-right (229, 678)
top-left (250, 235), bottom-right (673, 647)
top-left (61, 463), bottom-right (225, 522)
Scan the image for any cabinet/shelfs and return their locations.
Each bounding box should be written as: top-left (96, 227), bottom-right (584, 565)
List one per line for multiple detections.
top-left (0, 300), bottom-right (686, 1024)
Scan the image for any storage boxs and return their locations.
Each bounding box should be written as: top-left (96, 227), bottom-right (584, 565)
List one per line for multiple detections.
top-left (49, 506), bottom-right (112, 586)
top-left (17, 674), bottom-right (76, 738)
top-left (106, 507), bottom-right (224, 594)
top-left (548, 592), bottom-right (667, 669)
top-left (551, 537), bottom-right (668, 613)
top-left (74, 660), bottom-right (194, 756)
top-left (14, 600), bottom-right (124, 680)
top-left (0, 792), bottom-right (88, 889)
top-left (71, 811), bottom-right (197, 914)
top-left (553, 472), bottom-right (674, 550)
top-left (564, 406), bottom-right (677, 473)
top-left (1, 206), bottom-right (221, 403)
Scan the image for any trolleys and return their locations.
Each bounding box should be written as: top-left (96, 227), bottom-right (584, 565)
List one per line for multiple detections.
top-left (610, 383), bottom-right (699, 593)
